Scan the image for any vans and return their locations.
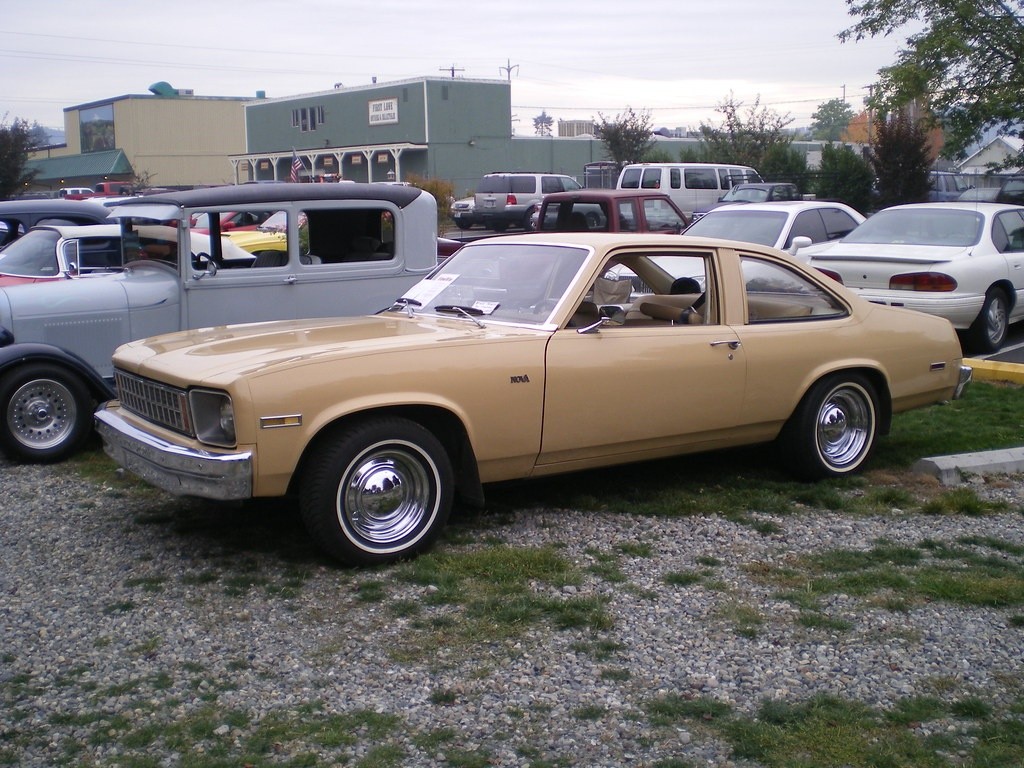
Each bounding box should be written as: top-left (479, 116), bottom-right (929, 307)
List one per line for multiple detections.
top-left (616, 163), bottom-right (768, 227)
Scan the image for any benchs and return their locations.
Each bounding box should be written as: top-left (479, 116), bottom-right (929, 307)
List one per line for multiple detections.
top-left (623, 293), bottom-right (814, 322)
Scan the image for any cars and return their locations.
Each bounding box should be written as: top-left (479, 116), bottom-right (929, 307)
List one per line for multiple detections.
top-left (89, 231), bottom-right (976, 564)
top-left (803, 201), bottom-right (1024, 353)
top-left (0, 194), bottom-right (310, 286)
top-left (0, 183), bottom-right (437, 465)
top-left (957, 188), bottom-right (1018, 206)
top-left (605, 200), bottom-right (868, 300)
top-left (449, 196), bottom-right (476, 230)
top-left (1004, 176), bottom-right (1024, 202)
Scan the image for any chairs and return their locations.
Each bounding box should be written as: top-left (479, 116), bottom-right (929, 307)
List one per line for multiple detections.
top-left (250, 249), bottom-right (288, 269)
top-left (563, 212), bottom-right (590, 232)
top-left (567, 301), bottom-right (599, 328)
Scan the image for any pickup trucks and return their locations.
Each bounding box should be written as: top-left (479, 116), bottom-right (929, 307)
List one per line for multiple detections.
top-left (532, 159), bottom-right (629, 225)
top-left (691, 182), bottom-right (839, 226)
top-left (64, 182), bottom-right (134, 200)
top-left (59, 188), bottom-right (94, 197)
top-left (437, 191), bottom-right (691, 266)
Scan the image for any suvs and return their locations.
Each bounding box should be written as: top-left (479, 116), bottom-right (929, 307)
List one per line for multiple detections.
top-left (474, 171), bottom-right (586, 229)
top-left (873, 172), bottom-right (973, 203)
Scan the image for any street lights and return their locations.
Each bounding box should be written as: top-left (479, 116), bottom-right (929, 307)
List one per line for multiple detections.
top-left (387, 169), bottom-right (394, 182)
